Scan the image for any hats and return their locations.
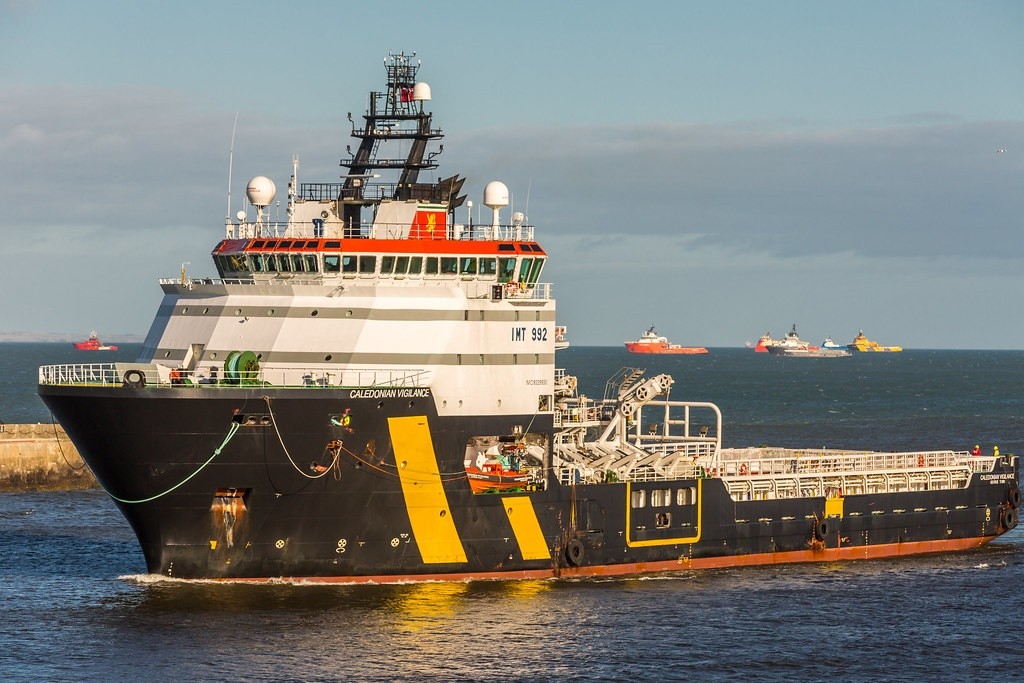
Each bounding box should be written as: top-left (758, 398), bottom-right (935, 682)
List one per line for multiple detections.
top-left (975, 445), bottom-right (979, 448)
top-left (994, 446), bottom-right (998, 449)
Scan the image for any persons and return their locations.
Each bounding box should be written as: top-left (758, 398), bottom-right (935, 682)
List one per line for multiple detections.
top-left (972, 445), bottom-right (982, 456)
top-left (993, 446), bottom-right (999, 456)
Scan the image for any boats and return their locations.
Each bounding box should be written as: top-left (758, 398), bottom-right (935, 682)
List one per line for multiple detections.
top-left (819, 336), bottom-right (849, 350)
top-left (38, 50), bottom-right (1020, 582)
top-left (464, 460), bottom-right (530, 493)
top-left (847, 329), bottom-right (903, 352)
top-left (72, 330), bottom-right (119, 351)
top-left (755, 323), bottom-right (819, 354)
top-left (765, 335), bottom-right (853, 358)
top-left (624, 324), bottom-right (708, 354)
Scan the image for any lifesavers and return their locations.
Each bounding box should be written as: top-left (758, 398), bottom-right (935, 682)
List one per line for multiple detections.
top-left (567, 539), bottom-right (585, 566)
top-left (815, 518), bottom-right (832, 540)
top-left (739, 463), bottom-right (748, 475)
top-left (1001, 486), bottom-right (1022, 530)
top-left (918, 455), bottom-right (925, 468)
top-left (504, 280), bottom-right (519, 294)
